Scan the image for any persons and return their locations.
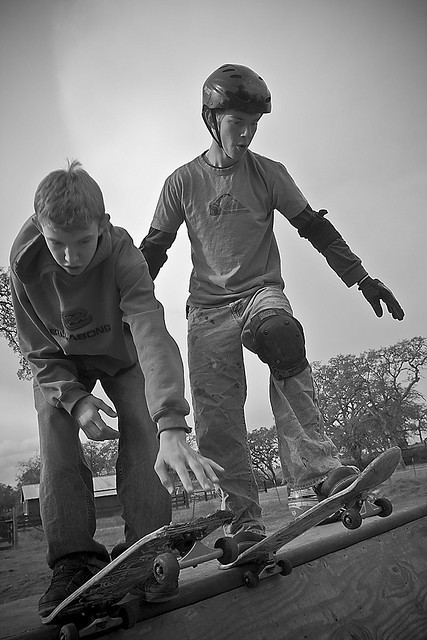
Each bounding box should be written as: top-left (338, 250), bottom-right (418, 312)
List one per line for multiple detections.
top-left (9, 158), bottom-right (225, 615)
top-left (138, 64), bottom-right (405, 555)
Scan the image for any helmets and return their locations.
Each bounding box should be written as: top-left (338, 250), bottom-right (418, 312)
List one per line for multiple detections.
top-left (202, 64), bottom-right (271, 117)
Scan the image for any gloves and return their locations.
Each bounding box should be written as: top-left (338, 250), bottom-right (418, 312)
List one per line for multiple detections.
top-left (358, 276), bottom-right (404, 320)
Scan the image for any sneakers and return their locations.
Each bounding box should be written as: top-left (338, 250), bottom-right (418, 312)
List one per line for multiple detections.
top-left (232, 532), bottom-right (266, 554)
top-left (312, 466), bottom-right (360, 502)
top-left (38, 553), bottom-right (93, 617)
top-left (129, 574), bottom-right (179, 603)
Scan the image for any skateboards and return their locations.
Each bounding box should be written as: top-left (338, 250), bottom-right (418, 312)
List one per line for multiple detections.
top-left (40, 510), bottom-right (239, 640)
top-left (217, 447), bottom-right (402, 587)
